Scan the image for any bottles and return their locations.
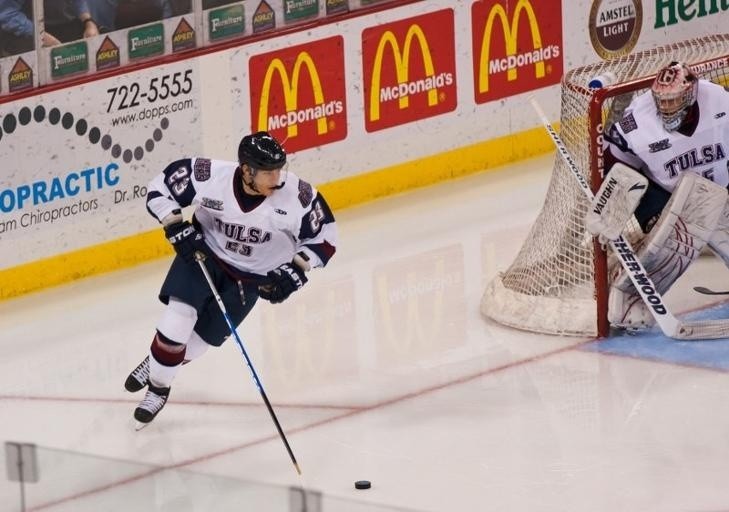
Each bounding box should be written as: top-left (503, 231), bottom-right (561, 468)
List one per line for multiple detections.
top-left (588, 70), bottom-right (617, 89)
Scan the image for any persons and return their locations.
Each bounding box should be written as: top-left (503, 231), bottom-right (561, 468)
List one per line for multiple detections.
top-left (124, 129), bottom-right (340, 422)
top-left (1, 0), bottom-right (101, 59)
top-left (601, 59), bottom-right (729, 335)
top-left (92, 0), bottom-right (173, 32)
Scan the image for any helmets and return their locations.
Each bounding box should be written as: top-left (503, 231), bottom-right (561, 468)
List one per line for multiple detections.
top-left (237, 131), bottom-right (287, 171)
top-left (651, 61), bottom-right (699, 135)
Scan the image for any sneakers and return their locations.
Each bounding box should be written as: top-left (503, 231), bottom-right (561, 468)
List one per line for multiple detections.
top-left (124, 354), bottom-right (151, 393)
top-left (134, 379), bottom-right (172, 424)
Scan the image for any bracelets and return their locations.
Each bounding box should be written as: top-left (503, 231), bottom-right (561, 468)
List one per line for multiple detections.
top-left (82, 17), bottom-right (97, 26)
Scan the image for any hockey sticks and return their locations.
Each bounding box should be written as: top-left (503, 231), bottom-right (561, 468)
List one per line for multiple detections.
top-left (693, 286), bottom-right (729, 295)
top-left (529, 99), bottom-right (729, 340)
top-left (193, 252), bottom-right (323, 492)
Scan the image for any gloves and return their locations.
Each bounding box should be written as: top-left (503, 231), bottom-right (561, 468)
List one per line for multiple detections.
top-left (258, 261), bottom-right (309, 305)
top-left (164, 216), bottom-right (205, 264)
